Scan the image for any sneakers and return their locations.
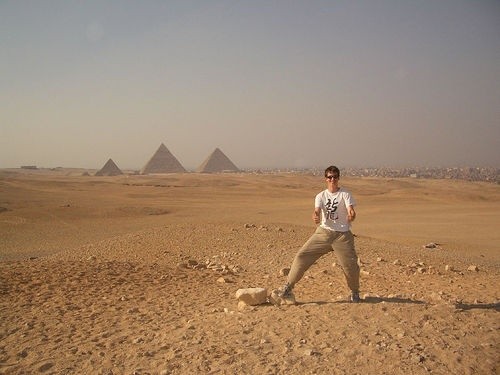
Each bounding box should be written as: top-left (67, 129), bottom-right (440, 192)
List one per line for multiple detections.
top-left (351, 293), bottom-right (360, 302)
top-left (278, 284), bottom-right (291, 297)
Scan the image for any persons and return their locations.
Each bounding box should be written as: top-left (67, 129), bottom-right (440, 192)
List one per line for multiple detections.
top-left (278, 166), bottom-right (360, 303)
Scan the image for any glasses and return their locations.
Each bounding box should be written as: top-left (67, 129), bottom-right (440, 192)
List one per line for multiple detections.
top-left (327, 176), bottom-right (339, 179)
